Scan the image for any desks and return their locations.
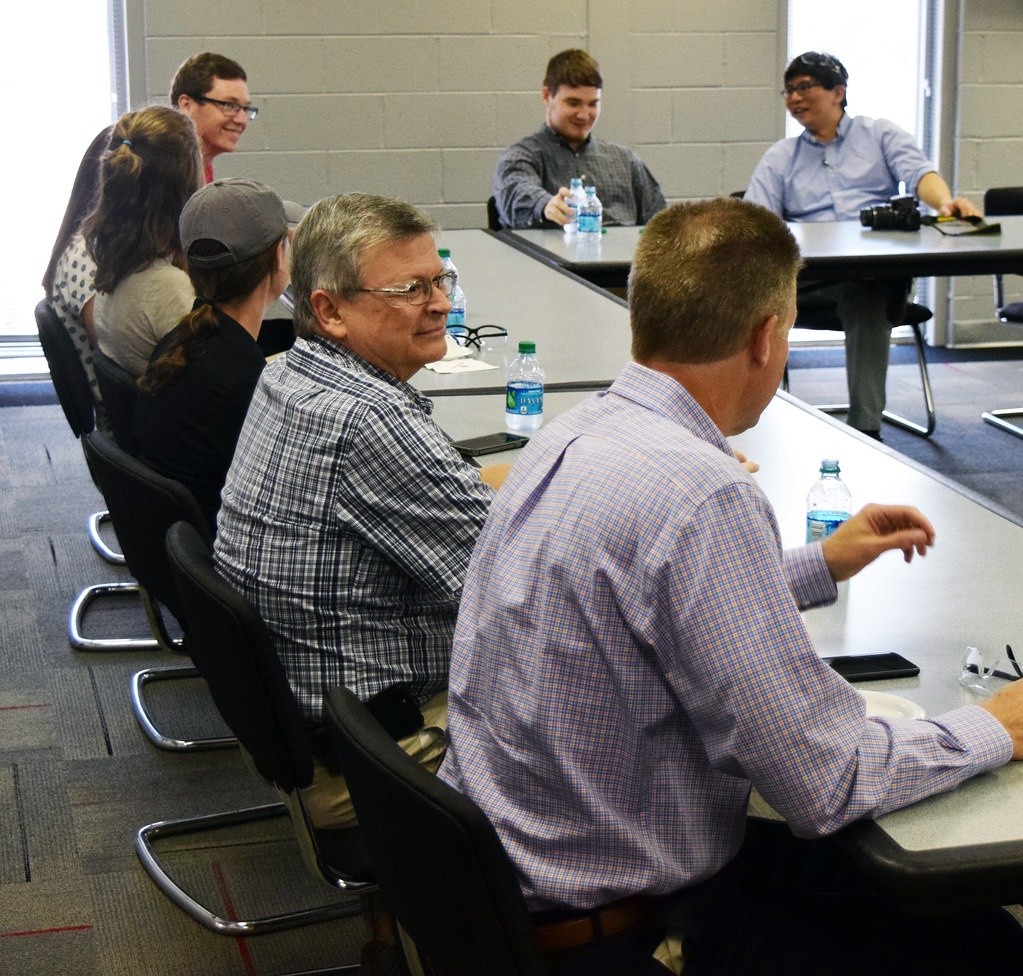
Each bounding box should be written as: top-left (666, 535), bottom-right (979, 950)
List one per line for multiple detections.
top-left (411, 377), bottom-right (1023, 907)
top-left (511, 214), bottom-right (1023, 298)
top-left (277, 226), bottom-right (645, 395)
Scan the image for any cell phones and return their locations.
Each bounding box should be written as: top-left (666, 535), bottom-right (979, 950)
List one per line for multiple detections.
top-left (821, 652), bottom-right (920, 681)
top-left (452, 432), bottom-right (529, 456)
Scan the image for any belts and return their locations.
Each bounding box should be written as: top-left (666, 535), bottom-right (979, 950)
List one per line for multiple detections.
top-left (539, 905), bottom-right (629, 952)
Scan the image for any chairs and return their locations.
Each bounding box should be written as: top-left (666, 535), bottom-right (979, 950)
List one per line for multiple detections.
top-left (35, 185), bottom-right (1023, 976)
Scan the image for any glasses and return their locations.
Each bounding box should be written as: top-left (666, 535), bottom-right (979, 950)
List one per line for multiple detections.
top-left (446, 324), bottom-right (508, 351)
top-left (781, 81), bottom-right (822, 96)
top-left (360, 271), bottom-right (456, 306)
top-left (961, 644), bottom-right (1022, 696)
top-left (190, 94), bottom-right (259, 122)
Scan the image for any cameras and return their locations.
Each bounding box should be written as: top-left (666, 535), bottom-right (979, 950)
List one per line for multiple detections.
top-left (861, 194), bottom-right (921, 232)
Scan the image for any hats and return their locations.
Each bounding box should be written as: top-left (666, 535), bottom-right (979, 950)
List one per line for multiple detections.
top-left (179, 175), bottom-right (307, 268)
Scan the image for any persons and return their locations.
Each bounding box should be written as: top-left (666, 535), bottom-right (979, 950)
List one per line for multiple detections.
top-left (41, 125), bottom-right (101, 398)
top-left (126, 178), bottom-right (307, 524)
top-left (209, 193), bottom-right (759, 976)
top-left (742, 51), bottom-right (984, 442)
top-left (493, 48), bottom-right (667, 229)
top-left (171, 52), bottom-right (258, 186)
top-left (434, 193), bottom-right (1023, 976)
top-left (82, 105), bottom-right (205, 376)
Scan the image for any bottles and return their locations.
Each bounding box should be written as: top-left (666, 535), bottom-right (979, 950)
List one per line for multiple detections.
top-left (437, 248), bottom-right (466, 333)
top-left (505, 340), bottom-right (545, 430)
top-left (576, 185), bottom-right (602, 243)
top-left (563, 178), bottom-right (585, 234)
top-left (806, 458), bottom-right (854, 543)
